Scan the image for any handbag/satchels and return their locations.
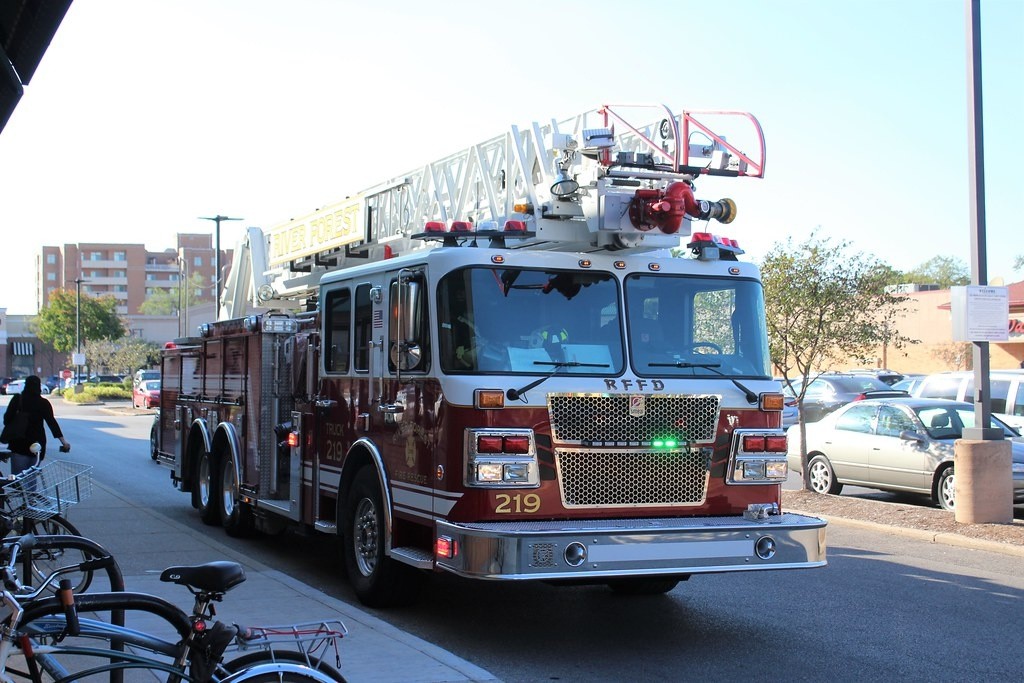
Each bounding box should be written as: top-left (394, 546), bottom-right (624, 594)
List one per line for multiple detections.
top-left (0, 394), bottom-right (21, 444)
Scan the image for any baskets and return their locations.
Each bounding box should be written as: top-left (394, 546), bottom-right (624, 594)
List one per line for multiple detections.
top-left (2, 460), bottom-right (93, 522)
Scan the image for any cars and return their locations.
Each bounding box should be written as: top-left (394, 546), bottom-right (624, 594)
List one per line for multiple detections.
top-left (47, 374), bottom-right (130, 391)
top-left (780, 376), bottom-right (911, 428)
top-left (890, 379), bottom-right (925, 396)
top-left (6, 380), bottom-right (50, 395)
top-left (134, 380), bottom-right (162, 409)
top-left (787, 397), bottom-right (1024, 511)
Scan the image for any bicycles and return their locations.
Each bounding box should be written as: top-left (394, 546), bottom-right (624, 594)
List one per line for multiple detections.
top-left (0, 535), bottom-right (352, 683)
top-left (0, 442), bottom-right (96, 593)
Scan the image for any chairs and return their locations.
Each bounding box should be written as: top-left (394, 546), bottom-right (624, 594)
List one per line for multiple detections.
top-left (925, 412), bottom-right (955, 438)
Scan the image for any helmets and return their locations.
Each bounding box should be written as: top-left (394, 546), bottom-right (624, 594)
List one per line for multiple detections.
top-left (528, 325), bottom-right (569, 355)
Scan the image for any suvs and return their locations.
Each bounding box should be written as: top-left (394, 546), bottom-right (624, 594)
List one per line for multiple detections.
top-left (845, 368), bottom-right (904, 385)
top-left (913, 370), bottom-right (1023, 435)
top-left (0, 377), bottom-right (18, 395)
top-left (133, 370), bottom-right (160, 389)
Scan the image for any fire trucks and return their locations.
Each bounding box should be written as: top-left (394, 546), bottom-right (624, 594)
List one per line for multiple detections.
top-left (156, 103), bottom-right (828, 609)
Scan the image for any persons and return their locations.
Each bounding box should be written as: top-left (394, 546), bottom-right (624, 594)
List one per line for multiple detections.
top-left (442, 285), bottom-right (521, 371)
top-left (3, 375), bottom-right (70, 507)
top-left (592, 294), bottom-right (673, 366)
